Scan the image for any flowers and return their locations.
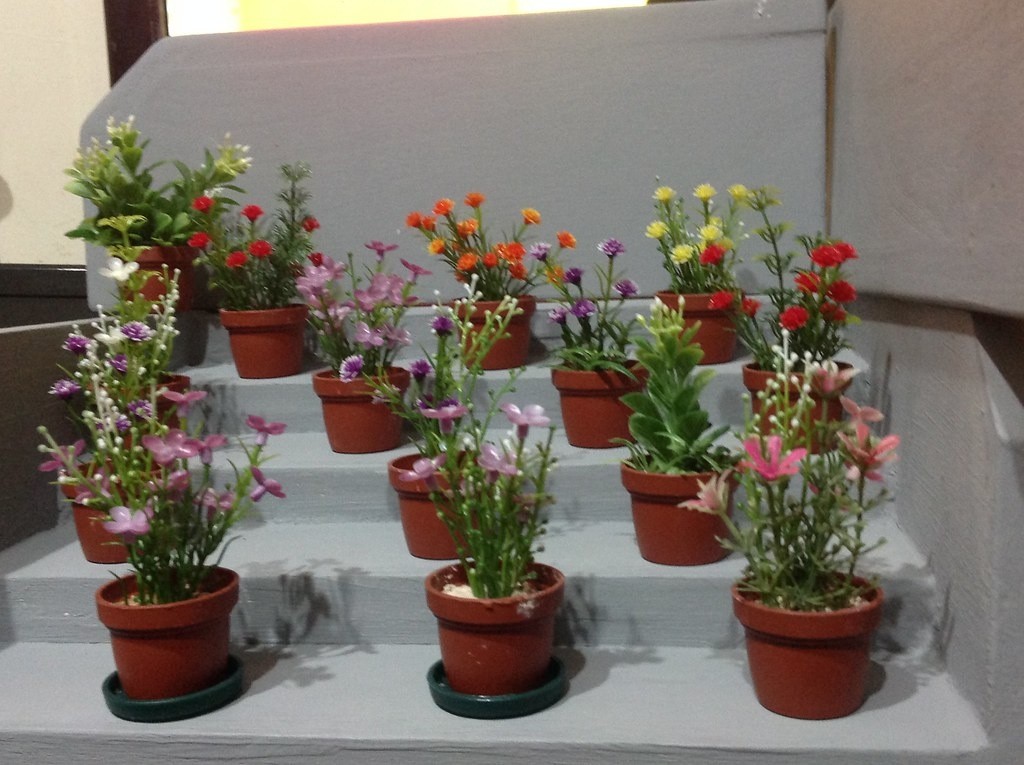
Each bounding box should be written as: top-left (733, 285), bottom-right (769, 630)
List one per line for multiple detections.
top-left (33, 109), bottom-right (901, 611)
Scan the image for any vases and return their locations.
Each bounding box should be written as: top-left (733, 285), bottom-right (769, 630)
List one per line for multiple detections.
top-left (216, 302), bottom-right (309, 378)
top-left (426, 559), bottom-right (566, 698)
top-left (732, 564), bottom-right (887, 722)
top-left (312, 364), bottom-right (411, 454)
top-left (656, 287), bottom-right (738, 364)
top-left (388, 451), bottom-right (497, 560)
top-left (620, 455), bottom-right (739, 568)
top-left (51, 461), bottom-right (178, 564)
top-left (741, 361), bottom-right (852, 456)
top-left (91, 565), bottom-right (242, 697)
top-left (87, 373), bottom-right (191, 451)
top-left (551, 362), bottom-right (649, 449)
top-left (454, 295), bottom-right (538, 372)
top-left (115, 243), bottom-right (208, 369)
